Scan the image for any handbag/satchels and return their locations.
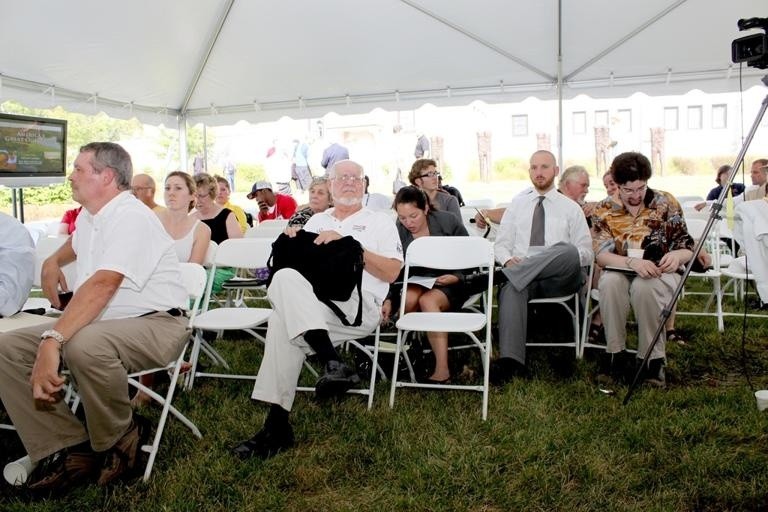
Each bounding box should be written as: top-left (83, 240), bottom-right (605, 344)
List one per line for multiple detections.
top-left (266, 229), bottom-right (366, 301)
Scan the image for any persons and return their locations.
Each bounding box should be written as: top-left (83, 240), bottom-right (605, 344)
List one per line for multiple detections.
top-left (707, 163), bottom-right (745, 200)
top-left (1, 135), bottom-right (713, 492)
top-left (696, 159), bottom-right (768, 212)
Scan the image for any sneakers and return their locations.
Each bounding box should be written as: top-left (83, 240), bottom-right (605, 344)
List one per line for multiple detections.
top-left (595, 350), bottom-right (623, 385)
top-left (231, 425), bottom-right (294, 461)
top-left (28, 442), bottom-right (98, 489)
top-left (643, 358), bottom-right (667, 386)
top-left (315, 361), bottom-right (361, 400)
top-left (98, 411), bottom-right (154, 485)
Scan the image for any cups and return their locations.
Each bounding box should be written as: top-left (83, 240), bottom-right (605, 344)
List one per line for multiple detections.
top-left (5, 450), bottom-right (43, 491)
top-left (626, 248), bottom-right (644, 269)
top-left (754, 390), bottom-right (768, 411)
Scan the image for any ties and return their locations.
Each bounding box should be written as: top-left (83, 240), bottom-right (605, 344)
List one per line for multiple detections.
top-left (530, 196), bottom-right (545, 246)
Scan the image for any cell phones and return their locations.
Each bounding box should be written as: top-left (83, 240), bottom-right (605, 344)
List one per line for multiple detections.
top-left (259, 200), bottom-right (268, 210)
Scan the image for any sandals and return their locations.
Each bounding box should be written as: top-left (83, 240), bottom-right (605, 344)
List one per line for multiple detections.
top-left (588, 323), bottom-right (605, 343)
top-left (666, 330), bottom-right (685, 344)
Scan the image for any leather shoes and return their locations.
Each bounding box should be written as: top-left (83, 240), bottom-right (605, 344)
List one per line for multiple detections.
top-left (465, 274), bottom-right (490, 296)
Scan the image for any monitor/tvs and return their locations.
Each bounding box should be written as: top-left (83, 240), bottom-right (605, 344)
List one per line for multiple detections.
top-left (0, 113), bottom-right (67, 178)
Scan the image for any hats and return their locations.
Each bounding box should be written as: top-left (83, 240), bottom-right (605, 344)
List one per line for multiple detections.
top-left (247, 180), bottom-right (273, 199)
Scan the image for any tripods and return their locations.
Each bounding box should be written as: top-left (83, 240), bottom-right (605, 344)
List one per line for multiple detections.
top-left (623, 93), bottom-right (768, 405)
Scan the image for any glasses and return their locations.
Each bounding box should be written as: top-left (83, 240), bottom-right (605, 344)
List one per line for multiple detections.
top-left (618, 184), bottom-right (648, 195)
top-left (312, 176), bottom-right (329, 182)
top-left (331, 175), bottom-right (367, 183)
top-left (419, 170), bottom-right (440, 177)
